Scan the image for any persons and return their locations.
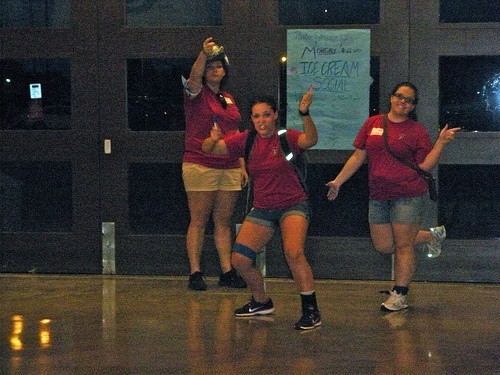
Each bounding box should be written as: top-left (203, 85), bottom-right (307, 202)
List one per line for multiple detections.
top-left (179, 36), bottom-right (254, 292)
top-left (321, 81), bottom-right (462, 318)
top-left (203, 82), bottom-right (327, 331)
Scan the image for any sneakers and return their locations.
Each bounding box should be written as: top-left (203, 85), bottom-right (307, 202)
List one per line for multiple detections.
top-left (427, 225), bottom-right (446, 258)
top-left (381, 289), bottom-right (409, 311)
top-left (293, 305), bottom-right (321, 329)
top-left (234, 295), bottom-right (275, 316)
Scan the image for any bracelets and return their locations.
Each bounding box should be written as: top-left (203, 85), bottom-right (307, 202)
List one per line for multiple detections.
top-left (298, 108), bottom-right (310, 117)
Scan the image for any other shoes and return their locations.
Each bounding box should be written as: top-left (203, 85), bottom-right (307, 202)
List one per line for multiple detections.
top-left (219, 274), bottom-right (248, 289)
top-left (188, 275), bottom-right (207, 291)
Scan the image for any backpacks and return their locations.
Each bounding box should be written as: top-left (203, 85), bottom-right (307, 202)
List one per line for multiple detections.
top-left (244, 127), bottom-right (308, 196)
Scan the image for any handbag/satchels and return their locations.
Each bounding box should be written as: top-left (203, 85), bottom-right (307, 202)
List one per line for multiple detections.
top-left (427, 171), bottom-right (438, 200)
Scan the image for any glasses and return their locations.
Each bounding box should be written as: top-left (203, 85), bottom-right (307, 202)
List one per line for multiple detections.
top-left (217, 93), bottom-right (228, 110)
top-left (393, 93), bottom-right (416, 104)
